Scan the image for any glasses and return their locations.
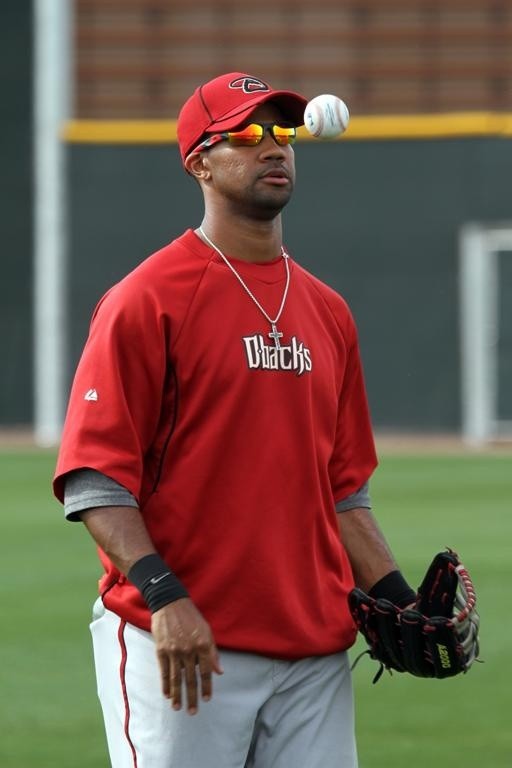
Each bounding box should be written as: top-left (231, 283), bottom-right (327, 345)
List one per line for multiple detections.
top-left (190, 119), bottom-right (296, 154)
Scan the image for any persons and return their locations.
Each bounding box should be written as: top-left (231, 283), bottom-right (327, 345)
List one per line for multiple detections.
top-left (48, 70), bottom-right (480, 768)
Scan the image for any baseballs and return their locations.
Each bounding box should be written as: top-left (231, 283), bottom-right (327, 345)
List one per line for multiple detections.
top-left (304, 94), bottom-right (349, 139)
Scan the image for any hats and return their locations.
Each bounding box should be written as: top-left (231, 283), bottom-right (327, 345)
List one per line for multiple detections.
top-left (177, 72), bottom-right (308, 176)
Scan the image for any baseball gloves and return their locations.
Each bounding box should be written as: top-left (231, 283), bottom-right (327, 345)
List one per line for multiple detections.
top-left (348, 547), bottom-right (479, 683)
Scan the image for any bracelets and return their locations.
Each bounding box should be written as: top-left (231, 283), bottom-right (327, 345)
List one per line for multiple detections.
top-left (366, 571), bottom-right (420, 612)
top-left (123, 552), bottom-right (191, 616)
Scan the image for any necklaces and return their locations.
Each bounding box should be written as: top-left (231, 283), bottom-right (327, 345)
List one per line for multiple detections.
top-left (194, 225), bottom-right (296, 351)
